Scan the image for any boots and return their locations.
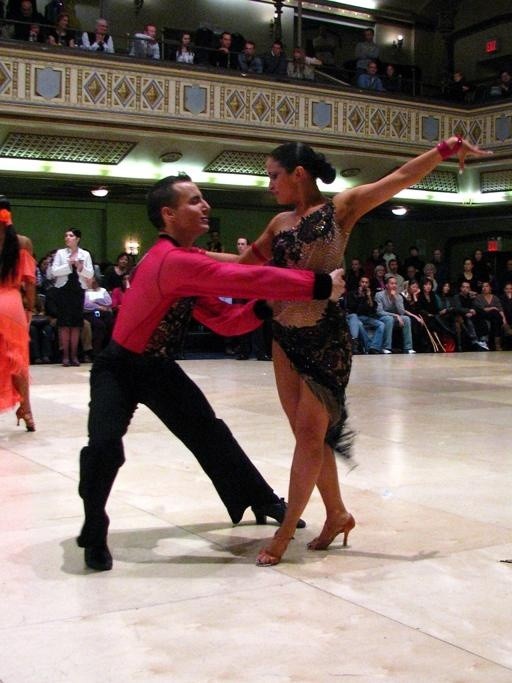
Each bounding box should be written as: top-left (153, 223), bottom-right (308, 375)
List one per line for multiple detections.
top-left (494, 337), bottom-right (503, 351)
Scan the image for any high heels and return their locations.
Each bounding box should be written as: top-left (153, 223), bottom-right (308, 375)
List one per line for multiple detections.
top-left (256, 530), bottom-right (294, 566)
top-left (84, 544), bottom-right (112, 570)
top-left (70, 352), bottom-right (81, 365)
top-left (308, 511), bottom-right (355, 550)
top-left (17, 406), bottom-right (36, 431)
top-left (63, 352), bottom-right (69, 365)
top-left (251, 495), bottom-right (305, 528)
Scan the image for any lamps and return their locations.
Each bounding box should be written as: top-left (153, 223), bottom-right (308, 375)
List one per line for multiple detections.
top-left (91, 185), bottom-right (109, 197)
top-left (390, 205), bottom-right (411, 217)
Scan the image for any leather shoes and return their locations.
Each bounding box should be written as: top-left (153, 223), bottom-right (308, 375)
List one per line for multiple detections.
top-left (352, 337), bottom-right (360, 345)
top-left (44, 356), bottom-right (51, 363)
top-left (368, 348), bottom-right (382, 354)
top-left (35, 358), bottom-right (42, 363)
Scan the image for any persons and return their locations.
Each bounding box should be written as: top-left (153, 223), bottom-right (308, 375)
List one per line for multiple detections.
top-left (187, 134), bottom-right (494, 565)
top-left (0, 217), bottom-right (511, 367)
top-left (77, 172), bottom-right (348, 571)
top-left (2, 1), bottom-right (511, 110)
top-left (1, 200), bottom-right (36, 431)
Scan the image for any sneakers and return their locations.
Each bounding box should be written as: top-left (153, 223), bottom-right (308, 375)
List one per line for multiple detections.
top-left (473, 340), bottom-right (490, 350)
top-left (403, 349), bottom-right (416, 354)
top-left (381, 348), bottom-right (392, 353)
